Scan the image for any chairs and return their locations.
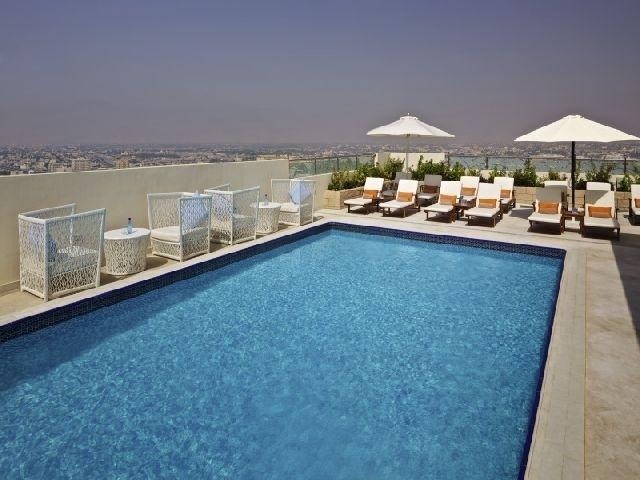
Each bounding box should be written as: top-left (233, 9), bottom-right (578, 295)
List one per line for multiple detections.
top-left (271, 178), bottom-right (320, 226)
top-left (18, 204), bottom-right (106, 302)
top-left (343, 172), bottom-right (640, 241)
top-left (147, 192), bottom-right (212, 262)
top-left (204, 184), bottom-right (260, 245)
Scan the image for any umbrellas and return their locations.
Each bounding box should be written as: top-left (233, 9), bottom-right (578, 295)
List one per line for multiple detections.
top-left (367, 111), bottom-right (456, 178)
top-left (513, 112), bottom-right (639, 216)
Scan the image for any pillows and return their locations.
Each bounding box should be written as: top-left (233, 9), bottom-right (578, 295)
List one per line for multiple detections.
top-left (175, 192), bottom-right (208, 228)
top-left (288, 178), bottom-right (312, 205)
top-left (208, 189), bottom-right (238, 222)
top-left (48, 235), bottom-right (57, 262)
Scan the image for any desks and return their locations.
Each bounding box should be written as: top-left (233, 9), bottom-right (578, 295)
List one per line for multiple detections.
top-left (249, 201), bottom-right (281, 234)
top-left (103, 228), bottom-right (151, 276)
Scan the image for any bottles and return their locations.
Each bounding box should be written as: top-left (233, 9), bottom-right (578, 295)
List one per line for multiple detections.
top-left (264, 193), bottom-right (269, 207)
top-left (127, 217), bottom-right (133, 235)
